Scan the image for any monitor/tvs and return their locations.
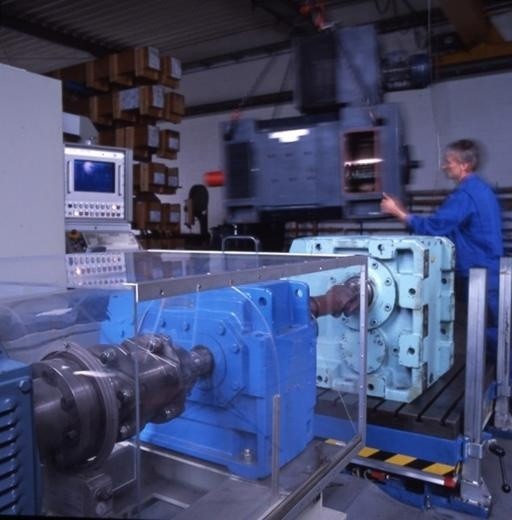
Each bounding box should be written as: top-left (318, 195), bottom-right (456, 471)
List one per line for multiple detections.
top-left (67, 153), bottom-right (119, 198)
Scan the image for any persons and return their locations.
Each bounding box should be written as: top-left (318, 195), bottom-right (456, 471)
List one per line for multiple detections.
top-left (380, 139), bottom-right (504, 364)
